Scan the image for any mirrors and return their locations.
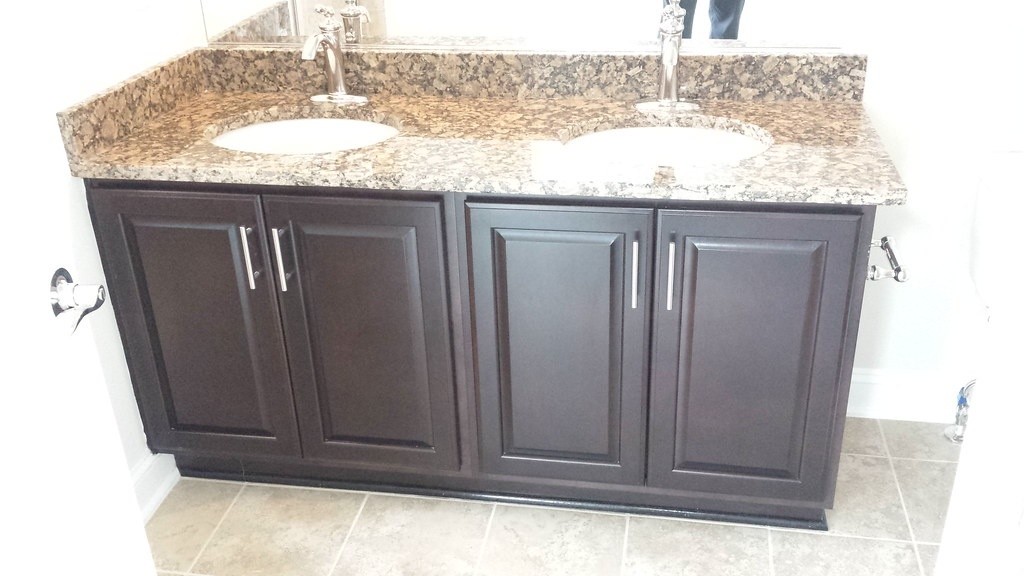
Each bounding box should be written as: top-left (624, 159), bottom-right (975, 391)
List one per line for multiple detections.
top-left (202, 0), bottom-right (838, 50)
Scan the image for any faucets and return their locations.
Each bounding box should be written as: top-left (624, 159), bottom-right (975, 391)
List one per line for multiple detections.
top-left (339, 0), bottom-right (371, 44)
top-left (633, 7), bottom-right (701, 112)
top-left (301, 5), bottom-right (370, 103)
top-left (657, 0), bottom-right (687, 44)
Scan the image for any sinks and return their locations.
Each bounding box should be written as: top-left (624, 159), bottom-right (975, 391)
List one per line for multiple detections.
top-left (209, 118), bottom-right (400, 155)
top-left (564, 126), bottom-right (771, 165)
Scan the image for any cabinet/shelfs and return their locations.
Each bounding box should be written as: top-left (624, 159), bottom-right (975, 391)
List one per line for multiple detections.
top-left (83, 176), bottom-right (877, 533)
